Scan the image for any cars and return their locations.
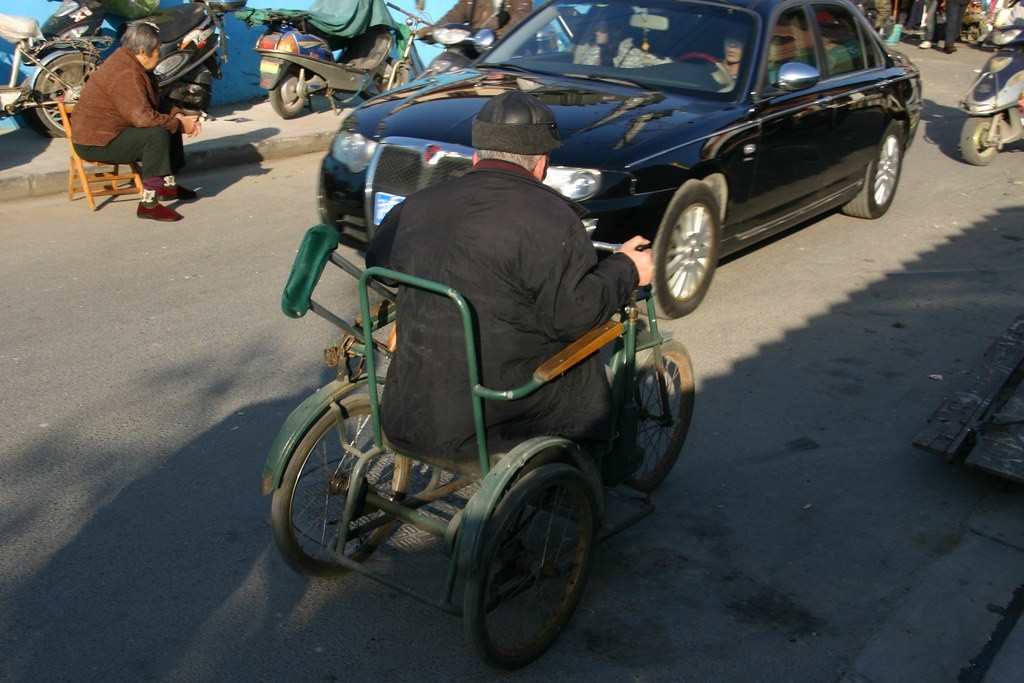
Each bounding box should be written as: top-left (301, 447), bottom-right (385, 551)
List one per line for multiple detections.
top-left (318, 1), bottom-right (923, 320)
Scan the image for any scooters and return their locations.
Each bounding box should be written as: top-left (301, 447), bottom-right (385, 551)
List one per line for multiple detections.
top-left (958, 25), bottom-right (1024, 167)
top-left (21, 0), bottom-right (247, 137)
top-left (414, 10), bottom-right (558, 77)
top-left (235, 1), bottom-right (409, 119)
top-left (936, 2), bottom-right (987, 47)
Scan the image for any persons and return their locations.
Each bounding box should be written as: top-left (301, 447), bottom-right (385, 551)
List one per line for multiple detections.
top-left (579, 7), bottom-right (663, 68)
top-left (70, 19), bottom-right (201, 222)
top-left (367, 90), bottom-right (656, 489)
top-left (914, 0), bottom-right (971, 53)
top-left (849, 0), bottom-right (892, 33)
top-left (694, 16), bottom-right (752, 91)
top-left (413, 0), bottom-right (532, 41)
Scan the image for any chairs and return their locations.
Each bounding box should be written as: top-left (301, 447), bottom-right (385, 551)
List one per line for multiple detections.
top-left (58, 103), bottom-right (144, 211)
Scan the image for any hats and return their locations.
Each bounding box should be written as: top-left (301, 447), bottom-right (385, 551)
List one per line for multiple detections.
top-left (472, 88), bottom-right (565, 154)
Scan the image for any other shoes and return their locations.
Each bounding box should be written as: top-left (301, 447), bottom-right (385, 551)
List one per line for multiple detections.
top-left (919, 40), bottom-right (938, 49)
top-left (136, 202), bottom-right (183, 221)
top-left (944, 42), bottom-right (957, 54)
top-left (156, 185), bottom-right (198, 200)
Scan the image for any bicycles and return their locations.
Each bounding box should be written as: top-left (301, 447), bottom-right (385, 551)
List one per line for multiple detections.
top-left (0, 13), bottom-right (115, 138)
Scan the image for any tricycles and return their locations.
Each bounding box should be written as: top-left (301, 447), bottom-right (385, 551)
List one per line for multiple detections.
top-left (262, 224), bottom-right (694, 671)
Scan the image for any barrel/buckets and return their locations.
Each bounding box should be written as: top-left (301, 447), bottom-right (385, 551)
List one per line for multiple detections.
top-left (881, 25), bottom-right (903, 45)
